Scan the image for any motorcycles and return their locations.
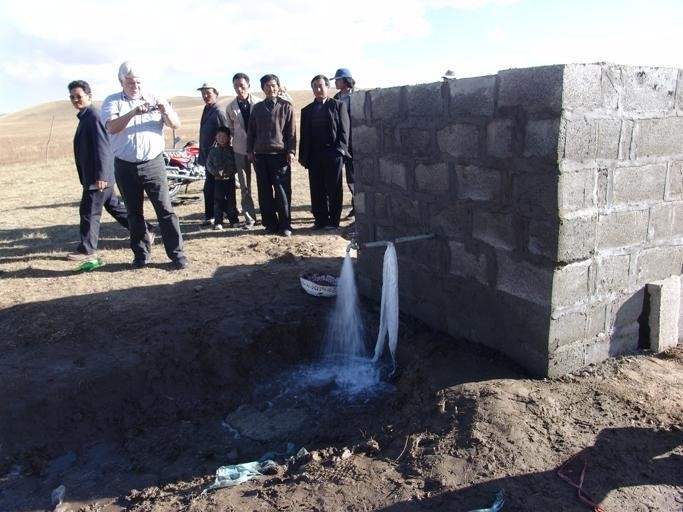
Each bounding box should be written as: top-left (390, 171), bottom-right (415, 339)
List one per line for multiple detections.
top-left (159, 138), bottom-right (249, 200)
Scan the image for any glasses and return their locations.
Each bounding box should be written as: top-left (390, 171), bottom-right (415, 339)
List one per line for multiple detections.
top-left (69, 94), bottom-right (83, 99)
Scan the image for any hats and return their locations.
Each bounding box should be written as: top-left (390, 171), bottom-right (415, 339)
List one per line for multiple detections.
top-left (196, 81), bottom-right (219, 93)
top-left (441, 69), bottom-right (457, 79)
top-left (329, 68), bottom-right (352, 81)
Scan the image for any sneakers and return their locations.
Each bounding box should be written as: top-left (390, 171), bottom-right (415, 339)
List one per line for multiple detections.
top-left (172, 255), bottom-right (190, 269)
top-left (66, 250), bottom-right (99, 261)
top-left (202, 214), bottom-right (293, 236)
top-left (311, 225), bottom-right (338, 231)
top-left (346, 210), bottom-right (355, 220)
top-left (148, 226), bottom-right (157, 245)
top-left (131, 259), bottom-right (150, 269)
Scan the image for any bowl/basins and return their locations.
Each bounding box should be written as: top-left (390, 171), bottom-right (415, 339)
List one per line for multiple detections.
top-left (298, 272), bottom-right (338, 299)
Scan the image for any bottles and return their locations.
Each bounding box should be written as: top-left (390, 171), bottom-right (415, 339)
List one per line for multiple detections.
top-left (71, 256), bottom-right (108, 275)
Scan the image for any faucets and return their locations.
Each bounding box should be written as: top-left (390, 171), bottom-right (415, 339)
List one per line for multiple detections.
top-left (345, 231), bottom-right (363, 253)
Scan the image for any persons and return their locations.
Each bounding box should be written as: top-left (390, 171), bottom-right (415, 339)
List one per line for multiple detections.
top-left (328, 68), bottom-right (359, 221)
top-left (226, 73), bottom-right (264, 230)
top-left (205, 126), bottom-right (241, 231)
top-left (100, 60), bottom-right (189, 270)
top-left (297, 74), bottom-right (351, 231)
top-left (246, 74), bottom-right (296, 237)
top-left (66, 79), bottom-right (158, 262)
top-left (196, 82), bottom-right (229, 227)
